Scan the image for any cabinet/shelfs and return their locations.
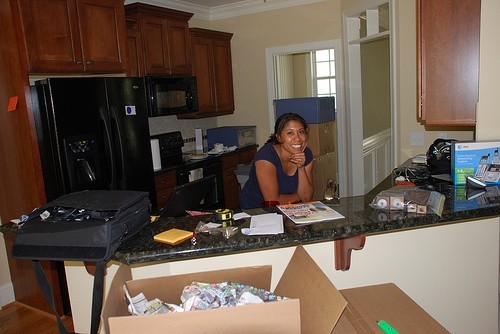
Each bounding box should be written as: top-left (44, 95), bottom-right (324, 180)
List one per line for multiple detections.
top-left (18, 0), bottom-right (128, 73)
top-left (416, 1), bottom-right (481, 126)
top-left (128, 30), bottom-right (145, 76)
top-left (222, 147), bottom-right (258, 210)
top-left (190, 36), bottom-right (234, 114)
top-left (153, 170), bottom-right (176, 211)
top-left (139, 12), bottom-right (189, 75)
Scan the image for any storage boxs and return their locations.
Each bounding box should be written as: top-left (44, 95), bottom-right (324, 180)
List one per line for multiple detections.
top-left (273, 97), bottom-right (336, 125)
top-left (206, 125), bottom-right (257, 150)
top-left (329, 283), bottom-right (452, 334)
top-left (101, 245), bottom-right (347, 334)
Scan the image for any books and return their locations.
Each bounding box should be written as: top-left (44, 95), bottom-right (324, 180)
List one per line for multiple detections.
top-left (250, 213), bottom-right (284, 235)
top-left (276, 201), bottom-right (346, 224)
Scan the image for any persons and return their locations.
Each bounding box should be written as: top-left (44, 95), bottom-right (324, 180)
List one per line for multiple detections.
top-left (239, 112), bottom-right (314, 209)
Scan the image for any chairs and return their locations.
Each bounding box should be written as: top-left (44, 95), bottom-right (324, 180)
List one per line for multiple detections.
top-left (163, 174), bottom-right (217, 218)
